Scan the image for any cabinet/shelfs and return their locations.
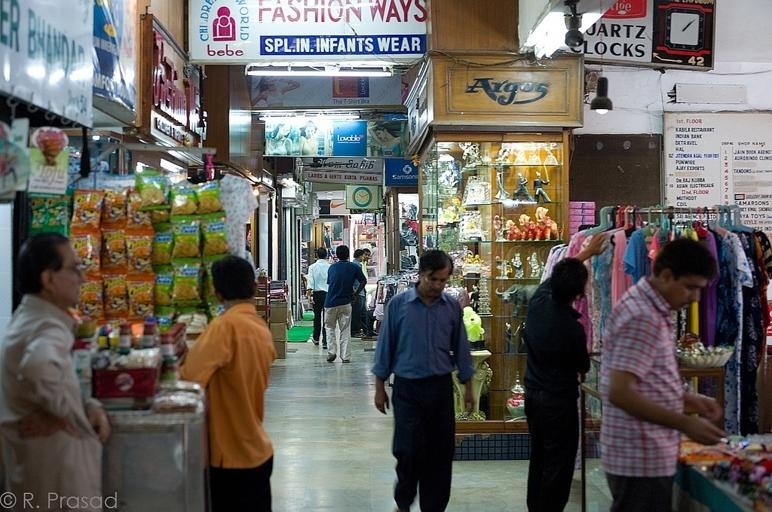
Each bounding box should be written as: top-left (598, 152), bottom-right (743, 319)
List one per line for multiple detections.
top-left (352, 214), bottom-right (384, 284)
top-left (403, 48), bottom-right (585, 432)
top-left (87, 382), bottom-right (211, 507)
top-left (667, 438), bottom-right (768, 511)
top-left (384, 186), bottom-right (419, 274)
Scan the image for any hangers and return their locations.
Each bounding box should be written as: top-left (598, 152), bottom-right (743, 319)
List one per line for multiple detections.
top-left (576, 206), bottom-right (758, 232)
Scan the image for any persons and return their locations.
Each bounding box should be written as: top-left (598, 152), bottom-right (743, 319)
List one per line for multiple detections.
top-left (298, 120), bottom-right (321, 156)
top-left (370, 248), bottom-right (477, 511)
top-left (267, 118), bottom-right (293, 156)
top-left (178, 256), bottom-right (277, 512)
top-left (324, 245), bottom-right (368, 363)
top-left (306, 247), bottom-right (332, 349)
top-left (352, 249), bottom-right (369, 337)
top-left (360, 248), bottom-right (370, 284)
top-left (0, 231), bottom-right (111, 512)
top-left (598, 235), bottom-right (726, 512)
top-left (368, 123), bottom-right (401, 156)
top-left (521, 230), bottom-right (613, 512)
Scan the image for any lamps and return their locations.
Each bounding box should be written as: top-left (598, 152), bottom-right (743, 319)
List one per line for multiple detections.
top-left (561, 1), bottom-right (590, 49)
top-left (590, 75), bottom-right (613, 116)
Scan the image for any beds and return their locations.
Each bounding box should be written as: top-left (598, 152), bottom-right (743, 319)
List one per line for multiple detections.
top-left (677, 363), bottom-right (736, 433)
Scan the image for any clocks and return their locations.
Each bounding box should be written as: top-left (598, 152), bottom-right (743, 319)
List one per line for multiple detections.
top-left (352, 186), bottom-right (373, 207)
top-left (652, 0), bottom-right (716, 67)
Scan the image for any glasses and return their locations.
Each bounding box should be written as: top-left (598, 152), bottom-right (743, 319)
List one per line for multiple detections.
top-left (425, 275), bottom-right (450, 284)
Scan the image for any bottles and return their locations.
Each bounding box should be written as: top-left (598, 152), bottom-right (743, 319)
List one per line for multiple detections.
top-left (140, 322), bottom-right (156, 349)
top-left (160, 322), bottom-right (190, 384)
top-left (117, 323), bottom-right (130, 354)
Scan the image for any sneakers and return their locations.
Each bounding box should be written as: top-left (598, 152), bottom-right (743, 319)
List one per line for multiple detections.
top-left (322, 344), bottom-right (327, 347)
top-left (327, 355), bottom-right (336, 361)
top-left (352, 332), bottom-right (363, 338)
top-left (362, 335), bottom-right (376, 340)
top-left (311, 338), bottom-right (319, 345)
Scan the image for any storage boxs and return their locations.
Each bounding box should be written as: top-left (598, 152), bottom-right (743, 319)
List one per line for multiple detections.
top-left (270, 301), bottom-right (288, 322)
top-left (268, 322), bottom-right (288, 342)
top-left (270, 342), bottom-right (286, 360)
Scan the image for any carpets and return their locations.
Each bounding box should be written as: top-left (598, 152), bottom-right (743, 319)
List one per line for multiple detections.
top-left (303, 310), bottom-right (316, 321)
top-left (287, 324), bottom-right (314, 343)
